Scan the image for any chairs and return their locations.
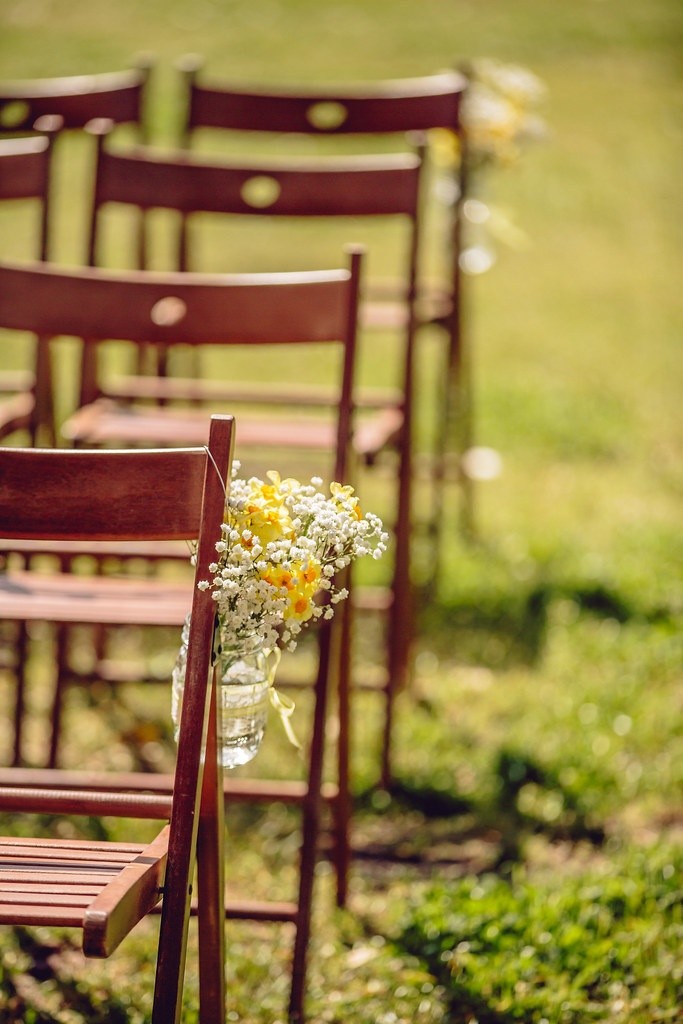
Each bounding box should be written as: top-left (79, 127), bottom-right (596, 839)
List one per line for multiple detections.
top-left (0, 413), bottom-right (236, 1024)
top-left (157, 62), bottom-right (474, 605)
top-left (1, 114), bottom-right (66, 765)
top-left (0, 243), bottom-right (364, 1024)
top-left (46, 120), bottom-right (433, 790)
top-left (0, 60), bottom-right (156, 405)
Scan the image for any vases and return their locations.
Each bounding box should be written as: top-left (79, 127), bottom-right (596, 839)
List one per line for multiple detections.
top-left (171, 611), bottom-right (270, 769)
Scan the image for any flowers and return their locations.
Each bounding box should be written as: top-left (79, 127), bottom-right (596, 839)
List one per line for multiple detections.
top-left (186, 459), bottom-right (390, 652)
